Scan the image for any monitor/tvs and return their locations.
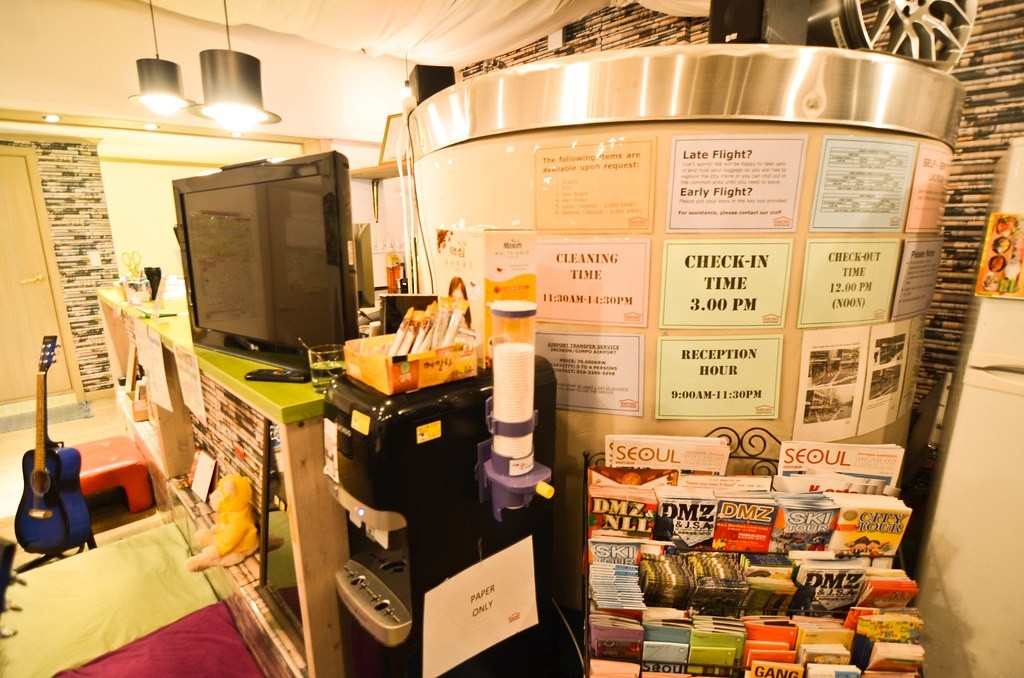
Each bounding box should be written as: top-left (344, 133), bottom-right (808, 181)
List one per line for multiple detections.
top-left (172, 150), bottom-right (359, 372)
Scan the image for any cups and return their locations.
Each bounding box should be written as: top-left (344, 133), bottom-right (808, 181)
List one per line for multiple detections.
top-left (308, 344), bottom-right (344, 394)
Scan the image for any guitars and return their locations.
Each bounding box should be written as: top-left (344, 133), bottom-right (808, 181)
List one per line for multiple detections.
top-left (13, 335), bottom-right (91, 554)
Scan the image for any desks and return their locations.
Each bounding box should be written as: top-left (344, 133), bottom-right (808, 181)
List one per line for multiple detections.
top-left (95, 278), bottom-right (346, 678)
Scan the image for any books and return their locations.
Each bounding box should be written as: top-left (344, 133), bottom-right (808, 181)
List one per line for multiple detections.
top-left (585, 432), bottom-right (926, 678)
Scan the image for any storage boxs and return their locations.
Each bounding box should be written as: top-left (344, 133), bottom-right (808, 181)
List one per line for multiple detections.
top-left (346, 332), bottom-right (479, 396)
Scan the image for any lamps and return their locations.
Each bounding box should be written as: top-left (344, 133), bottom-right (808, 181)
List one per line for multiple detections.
top-left (131, 0), bottom-right (194, 112)
top-left (199, 0), bottom-right (283, 127)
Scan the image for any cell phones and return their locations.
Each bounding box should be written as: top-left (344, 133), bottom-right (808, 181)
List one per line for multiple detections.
top-left (245, 369), bottom-right (311, 383)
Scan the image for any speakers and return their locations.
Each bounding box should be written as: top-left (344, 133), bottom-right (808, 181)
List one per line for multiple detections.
top-left (409, 65), bottom-right (456, 106)
top-left (708, 0), bottom-right (809, 45)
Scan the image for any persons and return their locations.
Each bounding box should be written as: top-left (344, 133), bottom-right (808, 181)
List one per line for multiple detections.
top-left (449, 277), bottom-right (473, 330)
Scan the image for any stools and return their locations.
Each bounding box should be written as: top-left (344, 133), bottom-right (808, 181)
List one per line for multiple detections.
top-left (75, 436), bottom-right (155, 522)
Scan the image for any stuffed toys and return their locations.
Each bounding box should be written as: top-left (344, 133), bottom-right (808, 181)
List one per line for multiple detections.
top-left (185, 476), bottom-right (258, 572)
